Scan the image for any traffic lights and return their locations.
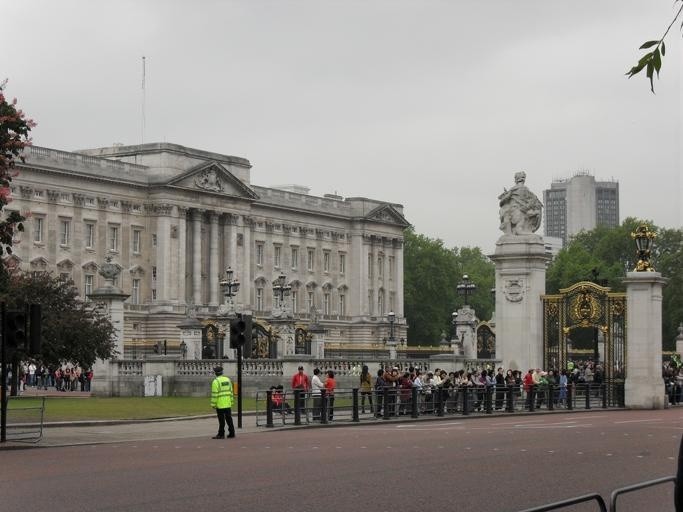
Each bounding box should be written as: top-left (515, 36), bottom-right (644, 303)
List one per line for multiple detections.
top-left (230, 319), bottom-right (246, 349)
top-left (0, 300), bottom-right (24, 364)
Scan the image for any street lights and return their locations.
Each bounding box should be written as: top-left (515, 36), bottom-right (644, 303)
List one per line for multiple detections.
top-left (219, 266), bottom-right (241, 305)
top-left (456, 274), bottom-right (476, 309)
top-left (181, 339), bottom-right (186, 376)
top-left (631, 219), bottom-right (658, 272)
top-left (386, 309), bottom-right (398, 341)
top-left (490, 288), bottom-right (495, 311)
top-left (382, 336), bottom-right (386, 350)
top-left (400, 337), bottom-right (404, 350)
top-left (272, 271), bottom-right (292, 309)
top-left (450, 311), bottom-right (459, 340)
top-left (579, 266), bottom-right (608, 397)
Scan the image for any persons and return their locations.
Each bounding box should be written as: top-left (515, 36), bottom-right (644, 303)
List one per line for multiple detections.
top-left (321, 370), bottom-right (337, 424)
top-left (270, 385), bottom-right (288, 415)
top-left (210, 365), bottom-right (236, 440)
top-left (0, 357), bottom-right (94, 392)
top-left (310, 368), bottom-right (327, 420)
top-left (277, 384), bottom-right (295, 415)
top-left (662, 357), bottom-right (682, 405)
top-left (359, 366), bottom-right (375, 413)
top-left (376, 365), bottom-right (568, 416)
top-left (566, 359), bottom-right (605, 397)
top-left (290, 365), bottom-right (310, 416)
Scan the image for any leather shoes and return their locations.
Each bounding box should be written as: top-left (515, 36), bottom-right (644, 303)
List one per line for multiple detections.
top-left (212, 435), bottom-right (225, 439)
top-left (227, 434), bottom-right (234, 438)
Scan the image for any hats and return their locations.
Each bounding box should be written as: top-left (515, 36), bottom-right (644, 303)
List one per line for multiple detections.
top-left (540, 371), bottom-right (548, 376)
top-left (528, 369), bottom-right (534, 371)
top-left (213, 366), bottom-right (224, 373)
top-left (298, 366), bottom-right (304, 370)
